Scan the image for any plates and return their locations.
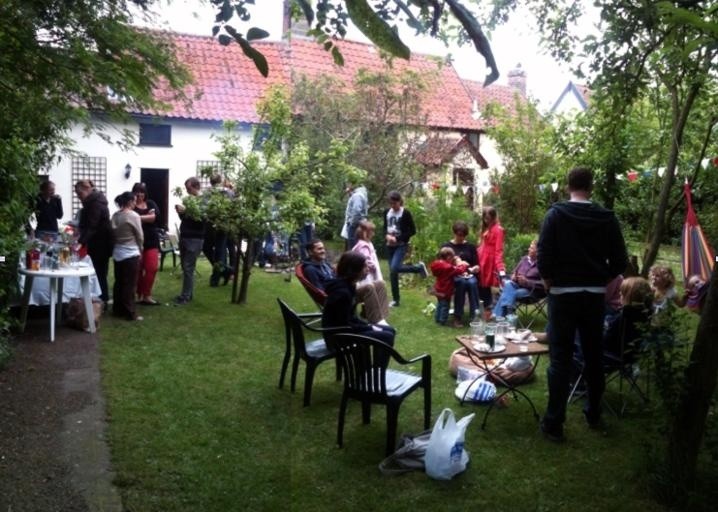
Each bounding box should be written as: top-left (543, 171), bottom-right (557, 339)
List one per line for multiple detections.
top-left (474, 342), bottom-right (506, 353)
top-left (503, 331), bottom-right (538, 342)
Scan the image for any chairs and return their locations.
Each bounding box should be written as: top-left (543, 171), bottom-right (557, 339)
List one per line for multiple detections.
top-left (157, 229), bottom-right (180, 273)
top-left (277, 297), bottom-right (352, 406)
top-left (516, 283), bottom-right (548, 329)
top-left (334, 333), bottom-right (432, 457)
top-left (568, 306), bottom-right (651, 418)
top-left (296, 264), bottom-right (329, 309)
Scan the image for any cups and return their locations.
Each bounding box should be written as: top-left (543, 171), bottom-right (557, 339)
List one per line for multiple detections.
top-left (498, 323), bottom-right (509, 345)
top-left (469, 322), bottom-right (482, 344)
top-left (518, 329), bottom-right (530, 351)
top-left (484, 324), bottom-right (496, 350)
top-left (493, 316), bottom-right (505, 334)
top-left (506, 313), bottom-right (518, 334)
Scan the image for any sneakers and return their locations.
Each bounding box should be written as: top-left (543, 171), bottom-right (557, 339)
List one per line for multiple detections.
top-left (420, 262), bottom-right (428, 278)
top-left (388, 301), bottom-right (400, 308)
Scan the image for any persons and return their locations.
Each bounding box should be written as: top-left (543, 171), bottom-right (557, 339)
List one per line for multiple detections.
top-left (572, 277), bottom-right (653, 394)
top-left (489, 240), bottom-right (549, 324)
top-left (348, 219), bottom-right (382, 285)
top-left (337, 175), bottom-right (370, 250)
top-left (109, 192), bottom-right (146, 321)
top-left (129, 183), bottom-right (162, 306)
top-left (431, 247), bottom-right (470, 325)
top-left (648, 264), bottom-right (692, 316)
top-left (171, 176), bottom-right (207, 306)
top-left (72, 179), bottom-right (114, 311)
top-left (382, 191), bottom-right (431, 307)
top-left (537, 167), bottom-right (629, 444)
top-left (440, 221), bottom-right (481, 328)
top-left (301, 238), bottom-right (391, 326)
top-left (320, 251), bottom-right (397, 368)
top-left (202, 173), bottom-right (235, 286)
top-left (34, 182), bottom-right (64, 242)
top-left (683, 273), bottom-right (709, 295)
top-left (477, 207), bottom-right (508, 310)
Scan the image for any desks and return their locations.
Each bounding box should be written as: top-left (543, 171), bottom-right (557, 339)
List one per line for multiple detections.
top-left (454, 332), bottom-right (548, 430)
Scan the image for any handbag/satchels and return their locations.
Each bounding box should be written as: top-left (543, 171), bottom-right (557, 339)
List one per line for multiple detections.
top-left (65, 297), bottom-right (105, 335)
top-left (379, 428), bottom-right (432, 475)
